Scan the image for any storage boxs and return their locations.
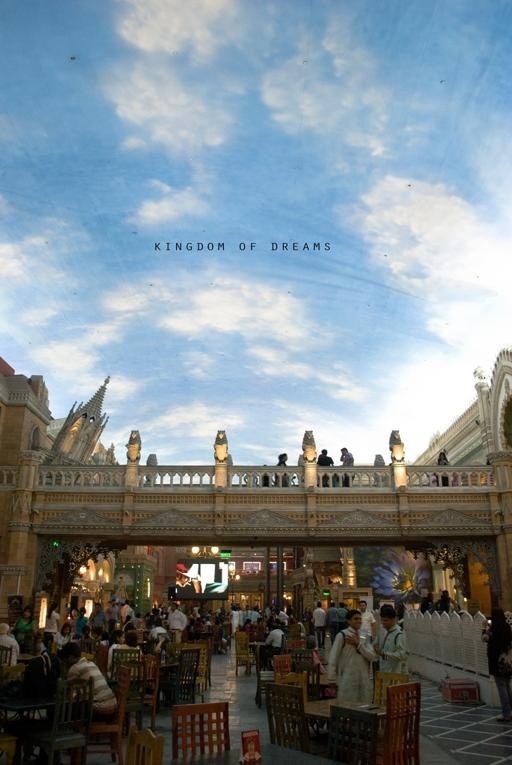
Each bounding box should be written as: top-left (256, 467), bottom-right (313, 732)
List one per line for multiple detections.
top-left (440, 678), bottom-right (480, 702)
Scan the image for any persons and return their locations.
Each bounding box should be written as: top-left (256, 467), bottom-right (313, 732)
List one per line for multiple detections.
top-left (421, 593), bottom-right (436, 616)
top-left (340, 448), bottom-right (354, 487)
top-left (274, 453), bottom-right (291, 487)
top-left (450, 471), bottom-right (459, 485)
top-left (176, 563), bottom-right (201, 595)
top-left (434, 452), bottom-right (451, 486)
top-left (317, 450), bottom-right (334, 487)
top-left (486, 607), bottom-right (511, 721)
top-left (434, 590), bottom-right (458, 615)
top-left (116, 577), bottom-right (128, 599)
top-left (262, 464), bottom-right (269, 487)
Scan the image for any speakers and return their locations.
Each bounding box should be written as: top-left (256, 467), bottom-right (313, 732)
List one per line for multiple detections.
top-left (168, 586), bottom-right (177, 600)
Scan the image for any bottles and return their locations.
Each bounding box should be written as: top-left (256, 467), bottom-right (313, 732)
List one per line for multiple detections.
top-left (248, 736), bottom-right (256, 760)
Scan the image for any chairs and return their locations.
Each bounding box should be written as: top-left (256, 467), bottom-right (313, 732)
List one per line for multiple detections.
top-left (1, 592), bottom-right (422, 765)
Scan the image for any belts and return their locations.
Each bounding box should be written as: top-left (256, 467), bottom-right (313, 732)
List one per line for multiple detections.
top-left (170, 629), bottom-right (179, 630)
